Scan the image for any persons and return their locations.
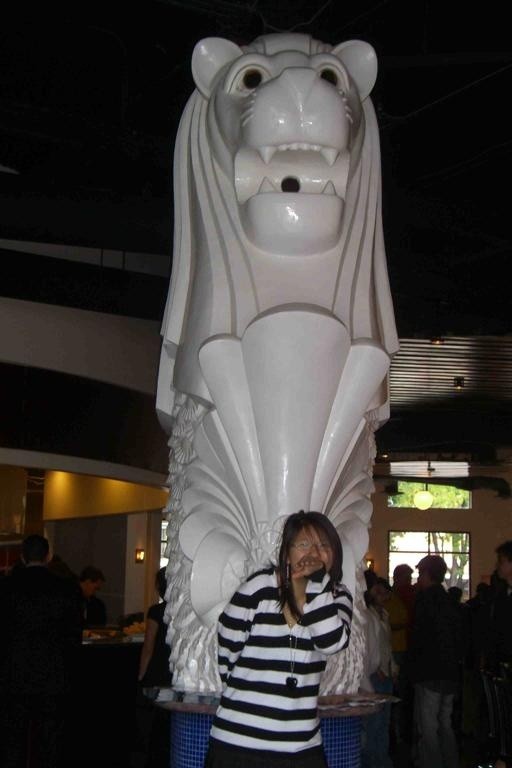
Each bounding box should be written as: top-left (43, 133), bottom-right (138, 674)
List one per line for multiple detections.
top-left (205, 509), bottom-right (353, 768)
top-left (359, 540), bottom-right (512, 768)
top-left (0, 534), bottom-right (171, 768)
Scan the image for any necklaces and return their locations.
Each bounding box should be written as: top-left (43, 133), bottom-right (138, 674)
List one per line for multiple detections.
top-left (286, 602), bottom-right (298, 689)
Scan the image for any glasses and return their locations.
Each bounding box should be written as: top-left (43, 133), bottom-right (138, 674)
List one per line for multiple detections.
top-left (291, 540), bottom-right (331, 550)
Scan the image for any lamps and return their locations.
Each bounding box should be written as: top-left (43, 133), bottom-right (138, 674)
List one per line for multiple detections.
top-left (412, 482), bottom-right (434, 511)
